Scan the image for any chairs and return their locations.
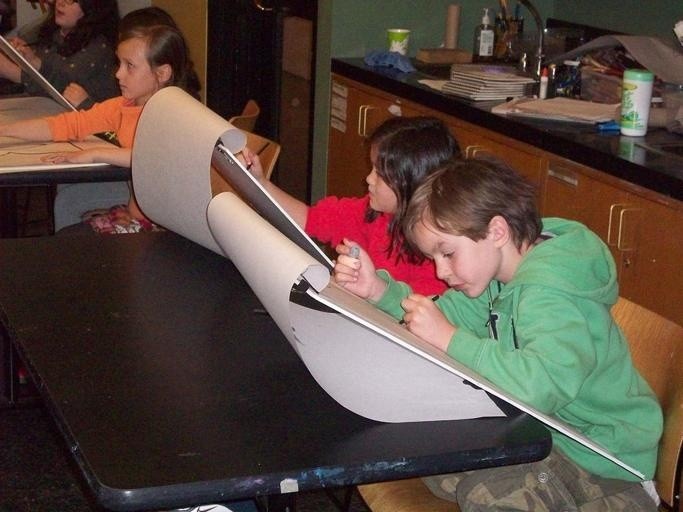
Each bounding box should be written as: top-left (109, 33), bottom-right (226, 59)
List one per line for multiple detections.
top-left (360, 295), bottom-right (683, 512)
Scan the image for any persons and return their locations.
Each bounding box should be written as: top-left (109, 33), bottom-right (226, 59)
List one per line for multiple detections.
top-left (1, 20), bottom-right (190, 234)
top-left (9, 4), bottom-right (202, 149)
top-left (332, 154), bottom-right (668, 512)
top-left (0, 1), bottom-right (123, 104)
top-left (238, 114), bottom-right (462, 299)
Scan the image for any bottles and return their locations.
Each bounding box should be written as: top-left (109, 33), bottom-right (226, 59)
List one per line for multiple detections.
top-left (619, 68), bottom-right (654, 137)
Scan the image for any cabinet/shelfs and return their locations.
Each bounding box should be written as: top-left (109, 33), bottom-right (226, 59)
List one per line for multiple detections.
top-left (324, 74), bottom-right (683, 326)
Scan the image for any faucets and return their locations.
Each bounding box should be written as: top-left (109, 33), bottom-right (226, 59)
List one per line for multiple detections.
top-left (518, 0), bottom-right (546, 76)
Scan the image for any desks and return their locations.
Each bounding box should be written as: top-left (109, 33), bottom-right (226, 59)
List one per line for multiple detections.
top-left (1, 230), bottom-right (551, 510)
top-left (2, 79), bottom-right (130, 240)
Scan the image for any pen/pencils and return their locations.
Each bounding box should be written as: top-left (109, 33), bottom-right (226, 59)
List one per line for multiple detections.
top-left (496, 0), bottom-right (523, 34)
top-left (247, 143), bottom-right (270, 170)
top-left (399, 295), bottom-right (439, 325)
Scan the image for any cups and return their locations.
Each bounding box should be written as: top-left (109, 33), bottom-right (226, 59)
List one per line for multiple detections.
top-left (386, 28), bottom-right (411, 56)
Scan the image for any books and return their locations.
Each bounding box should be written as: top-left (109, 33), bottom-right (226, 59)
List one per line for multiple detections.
top-left (416, 46), bottom-right (619, 126)
